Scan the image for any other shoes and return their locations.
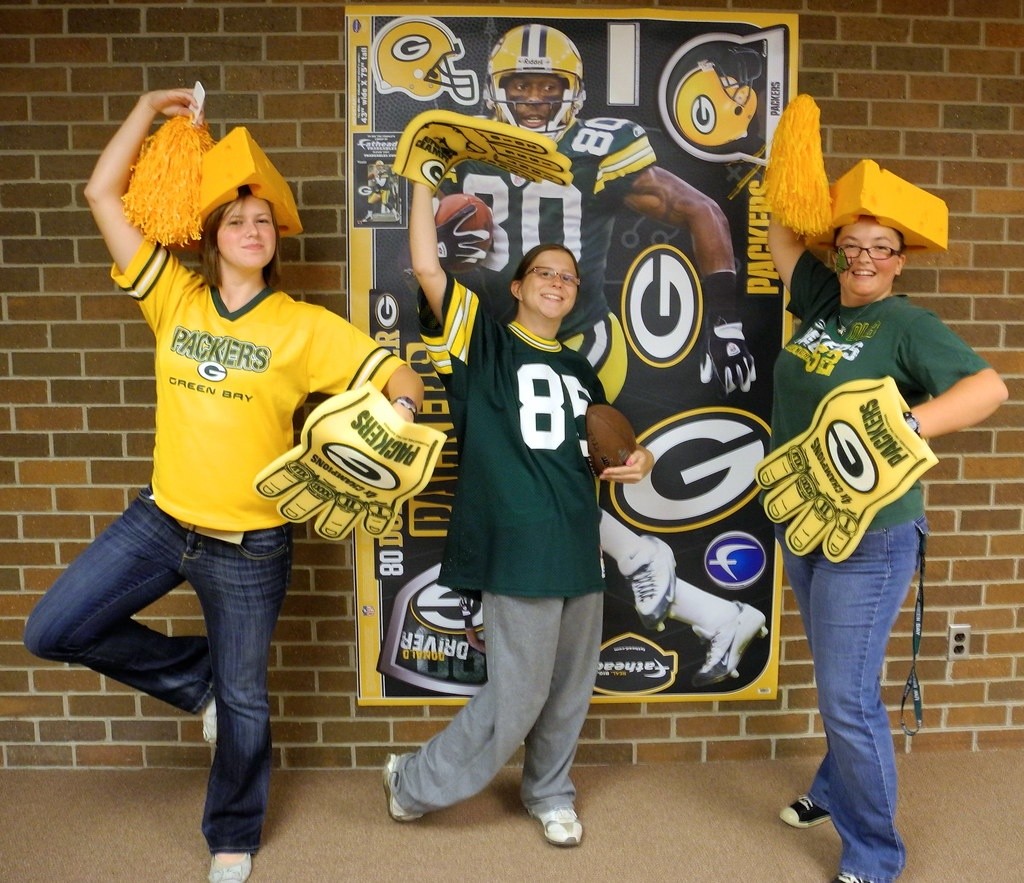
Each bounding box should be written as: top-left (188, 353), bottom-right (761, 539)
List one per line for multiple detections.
top-left (202, 696), bottom-right (217, 744)
top-left (208, 852), bottom-right (253, 883)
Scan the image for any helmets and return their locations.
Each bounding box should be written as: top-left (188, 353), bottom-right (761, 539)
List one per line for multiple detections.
top-left (486, 24), bottom-right (584, 143)
top-left (375, 160), bottom-right (384, 166)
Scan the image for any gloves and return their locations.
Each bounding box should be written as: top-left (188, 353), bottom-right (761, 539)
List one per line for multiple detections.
top-left (700, 308), bottom-right (756, 393)
top-left (431, 197), bottom-right (490, 270)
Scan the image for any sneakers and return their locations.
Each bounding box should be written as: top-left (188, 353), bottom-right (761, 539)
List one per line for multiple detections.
top-left (617, 535), bottom-right (677, 632)
top-left (830, 873), bottom-right (872, 883)
top-left (527, 805), bottom-right (583, 845)
top-left (779, 794), bottom-right (832, 828)
top-left (692, 600), bottom-right (768, 688)
top-left (395, 213), bottom-right (400, 222)
top-left (383, 753), bottom-right (423, 822)
top-left (362, 216), bottom-right (373, 222)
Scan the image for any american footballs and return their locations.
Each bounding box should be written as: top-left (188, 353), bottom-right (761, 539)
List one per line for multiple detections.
top-left (434, 193), bottom-right (494, 275)
top-left (585, 402), bottom-right (637, 473)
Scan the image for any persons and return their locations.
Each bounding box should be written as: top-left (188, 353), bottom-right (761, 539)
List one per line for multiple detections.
top-left (381, 109), bottom-right (655, 844)
top-left (359, 160), bottom-right (400, 222)
top-left (756, 94), bottom-right (1010, 883)
top-left (435, 22), bottom-right (770, 687)
top-left (25, 88), bottom-right (424, 882)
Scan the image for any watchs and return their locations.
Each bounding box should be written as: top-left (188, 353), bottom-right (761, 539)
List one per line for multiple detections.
top-left (391, 397), bottom-right (417, 422)
top-left (903, 412), bottom-right (919, 436)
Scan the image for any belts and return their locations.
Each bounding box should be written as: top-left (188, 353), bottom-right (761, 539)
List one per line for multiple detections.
top-left (176, 519), bottom-right (245, 545)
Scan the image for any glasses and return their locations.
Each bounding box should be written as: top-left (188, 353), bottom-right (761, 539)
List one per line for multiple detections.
top-left (522, 266), bottom-right (580, 287)
top-left (834, 243), bottom-right (901, 260)
